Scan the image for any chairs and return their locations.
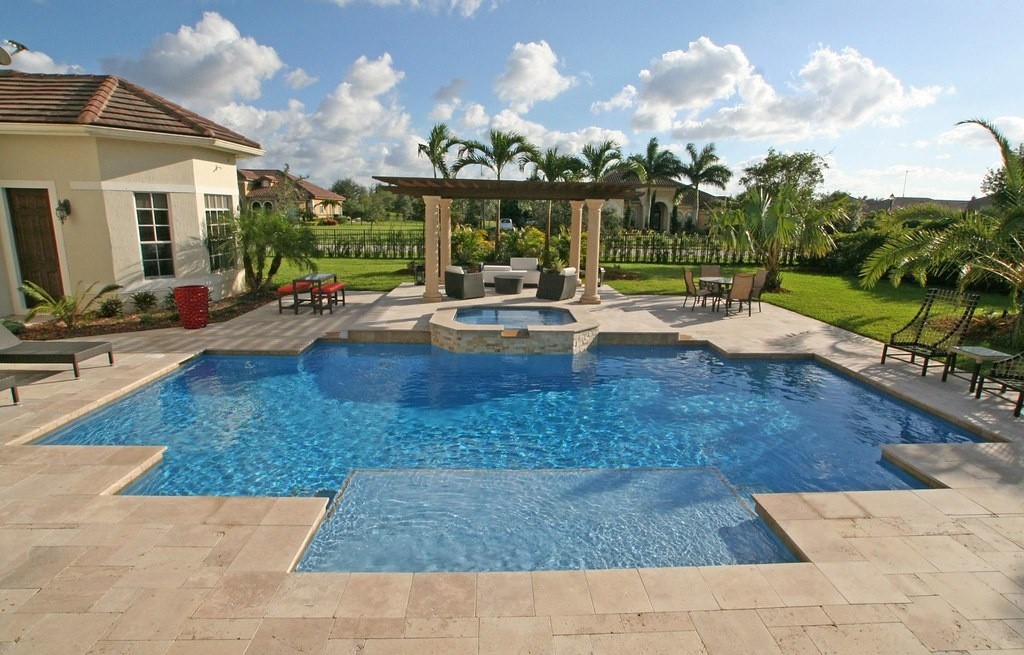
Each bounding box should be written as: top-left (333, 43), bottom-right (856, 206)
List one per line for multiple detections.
top-left (697, 264), bottom-right (724, 306)
top-left (975, 350), bottom-right (1024, 417)
top-left (682, 267), bottom-right (720, 312)
top-left (729, 269), bottom-right (769, 313)
top-left (717, 273), bottom-right (757, 317)
top-left (881, 287), bottom-right (980, 377)
top-left (0, 323), bottom-right (114, 403)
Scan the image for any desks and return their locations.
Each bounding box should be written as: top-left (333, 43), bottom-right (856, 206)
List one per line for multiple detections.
top-left (941, 346), bottom-right (1013, 393)
top-left (699, 277), bottom-right (745, 312)
top-left (494, 275), bottom-right (525, 294)
top-left (292, 273), bottom-right (338, 315)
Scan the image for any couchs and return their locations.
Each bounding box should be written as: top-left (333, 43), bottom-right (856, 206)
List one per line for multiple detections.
top-left (536, 272), bottom-right (579, 300)
top-left (447, 257), bottom-right (541, 288)
top-left (444, 270), bottom-right (486, 300)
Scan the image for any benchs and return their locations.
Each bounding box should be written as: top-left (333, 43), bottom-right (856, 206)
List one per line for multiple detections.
top-left (278, 282), bottom-right (314, 314)
top-left (311, 282), bottom-right (347, 314)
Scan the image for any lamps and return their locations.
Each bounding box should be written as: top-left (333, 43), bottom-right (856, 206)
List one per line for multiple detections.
top-left (56, 198), bottom-right (71, 226)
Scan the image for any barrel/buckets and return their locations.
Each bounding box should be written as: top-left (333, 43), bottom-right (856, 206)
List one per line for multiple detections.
top-left (174, 285), bottom-right (208, 329)
